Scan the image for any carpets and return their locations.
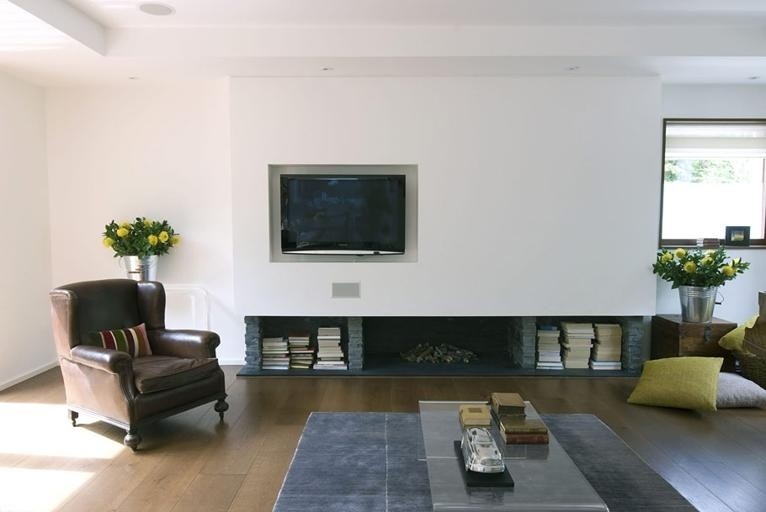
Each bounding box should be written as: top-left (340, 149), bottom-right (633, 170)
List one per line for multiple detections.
top-left (272, 413), bottom-right (698, 512)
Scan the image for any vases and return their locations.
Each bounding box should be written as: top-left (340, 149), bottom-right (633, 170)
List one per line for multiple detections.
top-left (131, 256), bottom-right (151, 282)
top-left (677, 285), bottom-right (719, 322)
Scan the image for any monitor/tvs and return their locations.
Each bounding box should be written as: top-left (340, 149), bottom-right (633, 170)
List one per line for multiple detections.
top-left (266, 163), bottom-right (418, 263)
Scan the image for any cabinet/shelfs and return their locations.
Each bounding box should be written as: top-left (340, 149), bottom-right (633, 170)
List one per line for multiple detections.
top-left (236, 315), bottom-right (648, 379)
top-left (654, 314), bottom-right (738, 374)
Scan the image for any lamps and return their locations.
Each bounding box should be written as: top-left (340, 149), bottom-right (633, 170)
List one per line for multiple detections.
top-left (140, 0), bottom-right (175, 18)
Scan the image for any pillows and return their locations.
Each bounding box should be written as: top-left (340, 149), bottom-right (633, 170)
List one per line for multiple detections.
top-left (628, 357), bottom-right (725, 412)
top-left (90, 323), bottom-right (151, 361)
top-left (713, 371), bottom-right (764, 411)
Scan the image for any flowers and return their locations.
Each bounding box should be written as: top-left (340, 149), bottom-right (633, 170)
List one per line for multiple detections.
top-left (651, 245), bottom-right (749, 286)
top-left (102, 217), bottom-right (182, 255)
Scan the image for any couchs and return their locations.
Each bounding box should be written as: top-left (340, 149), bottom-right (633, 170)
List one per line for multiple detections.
top-left (48, 280), bottom-right (229, 453)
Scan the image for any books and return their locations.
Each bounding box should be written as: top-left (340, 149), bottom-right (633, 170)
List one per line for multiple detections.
top-left (262, 334), bottom-right (290, 370)
top-left (560, 320), bottom-right (595, 368)
top-left (535, 326), bottom-right (564, 371)
top-left (589, 322), bottom-right (623, 371)
top-left (457, 402), bottom-right (491, 424)
top-left (288, 323), bottom-right (313, 368)
top-left (313, 325), bottom-right (347, 370)
top-left (490, 391), bottom-right (550, 445)
